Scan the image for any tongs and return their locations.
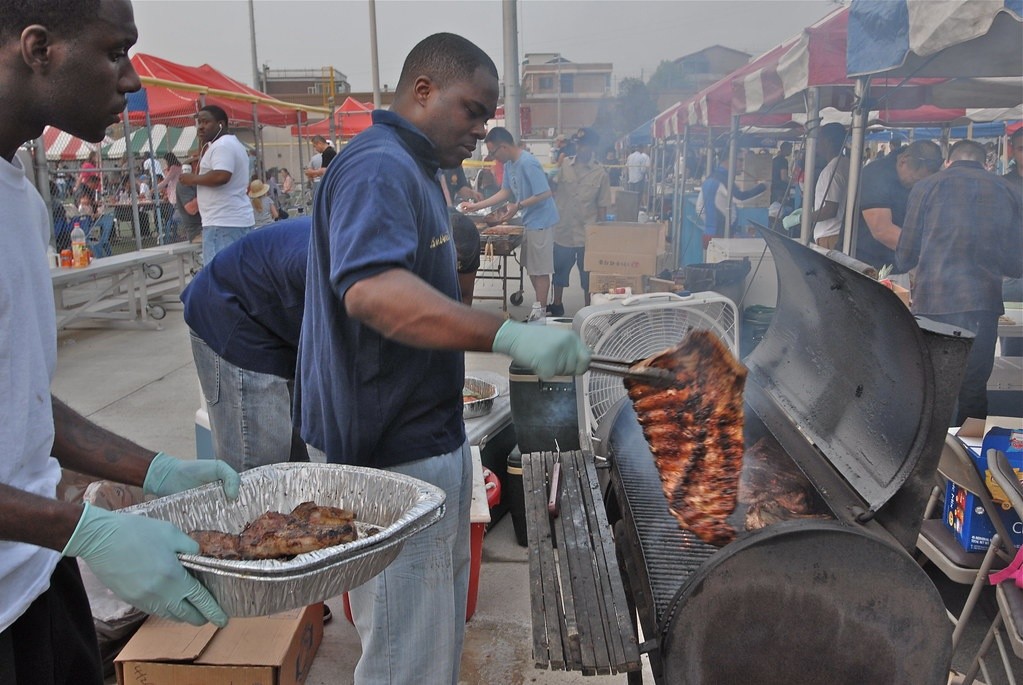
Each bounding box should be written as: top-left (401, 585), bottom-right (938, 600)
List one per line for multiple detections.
top-left (588, 355), bottom-right (676, 387)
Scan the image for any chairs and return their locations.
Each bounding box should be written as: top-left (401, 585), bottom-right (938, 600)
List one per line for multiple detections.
top-left (915, 432), bottom-right (1023, 685)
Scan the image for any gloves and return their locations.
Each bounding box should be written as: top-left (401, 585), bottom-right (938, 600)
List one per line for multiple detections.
top-left (783, 208), bottom-right (802, 230)
top-left (142, 451), bottom-right (241, 500)
top-left (491, 317), bottom-right (592, 380)
top-left (62, 499), bottom-right (230, 628)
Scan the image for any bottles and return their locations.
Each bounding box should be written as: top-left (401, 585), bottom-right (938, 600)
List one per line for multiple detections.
top-left (71, 223), bottom-right (85, 266)
top-left (672, 177), bottom-right (700, 191)
top-left (47, 247), bottom-right (61, 269)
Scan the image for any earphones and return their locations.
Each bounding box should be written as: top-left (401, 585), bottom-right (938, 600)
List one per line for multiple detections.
top-left (219, 123), bottom-right (222, 129)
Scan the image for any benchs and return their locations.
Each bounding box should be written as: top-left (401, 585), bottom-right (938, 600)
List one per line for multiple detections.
top-left (52, 240), bottom-right (202, 337)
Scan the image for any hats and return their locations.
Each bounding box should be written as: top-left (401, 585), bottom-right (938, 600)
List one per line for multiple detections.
top-left (139, 174), bottom-right (150, 181)
top-left (325, 140), bottom-right (334, 147)
top-left (780, 142), bottom-right (793, 150)
top-left (571, 127), bottom-right (599, 146)
top-left (248, 179), bottom-right (269, 198)
top-left (553, 134), bottom-right (567, 141)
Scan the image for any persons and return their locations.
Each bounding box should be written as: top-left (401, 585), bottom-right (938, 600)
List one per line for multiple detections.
top-left (42, 122), bottom-right (1019, 423)
top-left (293, 33), bottom-right (592, 685)
top-left (178, 215), bottom-right (315, 477)
top-left (0, 0), bottom-right (240, 685)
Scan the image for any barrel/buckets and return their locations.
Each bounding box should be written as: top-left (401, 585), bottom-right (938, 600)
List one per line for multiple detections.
top-left (742, 311), bottom-right (775, 362)
top-left (342, 465), bottom-right (501, 627)
top-left (686, 262), bottom-right (745, 343)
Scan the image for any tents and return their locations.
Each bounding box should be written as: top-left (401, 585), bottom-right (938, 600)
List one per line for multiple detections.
top-left (610, 131), bottom-right (636, 184)
top-left (660, 76), bottom-right (726, 223)
top-left (839, 0), bottom-right (1023, 259)
top-left (195, 63), bottom-right (317, 216)
top-left (291, 96), bottom-right (379, 209)
top-left (34, 121), bottom-right (117, 205)
top-left (722, 3), bottom-right (854, 248)
top-left (627, 100), bottom-right (685, 209)
top-left (676, 36), bottom-right (790, 268)
top-left (107, 118), bottom-right (204, 163)
top-left (109, 52), bottom-right (273, 246)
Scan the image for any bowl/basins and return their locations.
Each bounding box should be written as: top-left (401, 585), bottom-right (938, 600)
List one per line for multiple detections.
top-left (137, 196), bottom-right (144, 202)
top-left (110, 199), bottom-right (117, 204)
top-left (108, 462), bottom-right (446, 619)
top-left (463, 377), bottom-right (499, 419)
top-left (485, 204), bottom-right (507, 227)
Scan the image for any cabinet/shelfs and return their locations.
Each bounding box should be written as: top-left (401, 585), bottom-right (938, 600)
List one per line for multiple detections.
top-left (657, 191), bottom-right (769, 273)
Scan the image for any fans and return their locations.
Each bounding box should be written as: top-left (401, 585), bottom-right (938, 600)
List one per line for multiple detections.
top-left (575, 290), bottom-right (739, 446)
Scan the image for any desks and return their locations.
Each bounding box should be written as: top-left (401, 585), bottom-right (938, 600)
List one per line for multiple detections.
top-left (997, 301), bottom-right (1023, 337)
top-left (103, 198), bottom-right (169, 240)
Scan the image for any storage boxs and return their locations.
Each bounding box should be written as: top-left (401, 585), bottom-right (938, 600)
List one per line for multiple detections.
top-left (583, 188), bottom-right (672, 300)
top-left (890, 283), bottom-right (911, 312)
top-left (733, 152), bottom-right (772, 208)
top-left (943, 415), bottom-right (1023, 550)
top-left (461, 360), bottom-right (618, 549)
top-left (113, 599), bottom-right (325, 685)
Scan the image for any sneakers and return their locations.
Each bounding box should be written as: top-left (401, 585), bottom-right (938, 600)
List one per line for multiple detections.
top-left (546, 303), bottom-right (564, 314)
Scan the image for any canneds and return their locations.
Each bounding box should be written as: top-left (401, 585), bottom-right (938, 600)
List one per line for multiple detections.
top-left (86, 248), bottom-right (90, 264)
top-left (60, 250), bottom-right (72, 268)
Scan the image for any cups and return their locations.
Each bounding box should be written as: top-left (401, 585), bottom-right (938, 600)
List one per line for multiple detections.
top-left (182, 165), bottom-right (192, 174)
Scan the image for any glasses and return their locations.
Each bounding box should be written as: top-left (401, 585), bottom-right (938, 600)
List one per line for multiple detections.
top-left (736, 157), bottom-right (744, 162)
top-left (488, 146), bottom-right (501, 160)
